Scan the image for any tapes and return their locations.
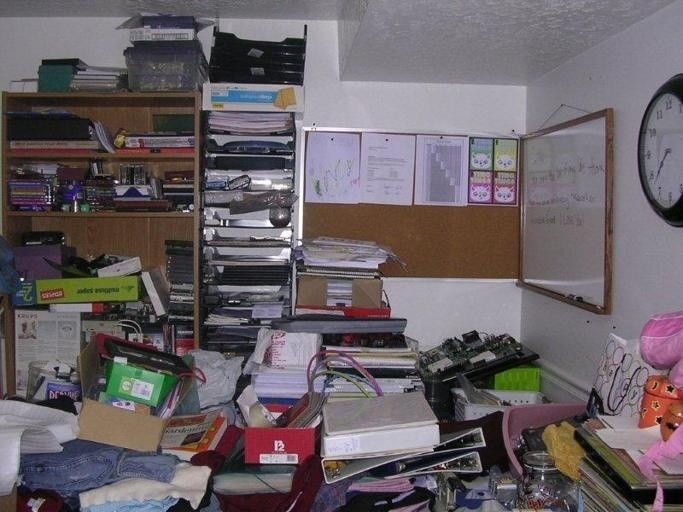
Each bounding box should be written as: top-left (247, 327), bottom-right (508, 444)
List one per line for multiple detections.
top-left (249, 401), bottom-right (278, 428)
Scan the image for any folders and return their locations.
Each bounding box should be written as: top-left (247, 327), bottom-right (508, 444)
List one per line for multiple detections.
top-left (320, 427), bottom-right (486, 485)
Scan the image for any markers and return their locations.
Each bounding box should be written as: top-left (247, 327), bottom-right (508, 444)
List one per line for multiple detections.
top-left (564, 292), bottom-right (583, 303)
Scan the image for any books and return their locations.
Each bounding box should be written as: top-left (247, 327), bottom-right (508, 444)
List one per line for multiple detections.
top-left (10, 140), bottom-right (102, 149)
top-left (1, 256), bottom-right (228, 461)
top-left (69, 70), bottom-right (129, 91)
top-left (295, 234), bottom-right (391, 318)
top-left (124, 136), bottom-right (196, 148)
top-left (250, 328), bottom-right (439, 436)
top-left (208, 110), bottom-right (294, 136)
top-left (565, 412), bottom-right (682, 512)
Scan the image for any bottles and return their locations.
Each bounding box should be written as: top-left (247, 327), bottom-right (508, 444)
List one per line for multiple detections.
top-left (660, 401), bottom-right (683, 441)
top-left (517, 449), bottom-right (583, 512)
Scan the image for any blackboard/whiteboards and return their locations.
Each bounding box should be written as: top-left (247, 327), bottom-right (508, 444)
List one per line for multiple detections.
top-left (516, 108), bottom-right (613, 315)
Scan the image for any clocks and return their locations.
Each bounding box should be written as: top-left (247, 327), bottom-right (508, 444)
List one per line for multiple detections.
top-left (637, 73), bottom-right (683, 228)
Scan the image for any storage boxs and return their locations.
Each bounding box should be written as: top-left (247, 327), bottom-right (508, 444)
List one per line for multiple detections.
top-left (295, 272), bottom-right (382, 308)
top-left (75, 335), bottom-right (185, 454)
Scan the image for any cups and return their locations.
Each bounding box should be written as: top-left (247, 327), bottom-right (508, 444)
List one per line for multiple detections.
top-left (71, 200), bottom-right (79, 213)
top-left (269, 206), bottom-right (291, 228)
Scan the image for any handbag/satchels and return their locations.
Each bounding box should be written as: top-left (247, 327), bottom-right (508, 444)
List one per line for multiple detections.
top-left (585, 331), bottom-right (669, 420)
